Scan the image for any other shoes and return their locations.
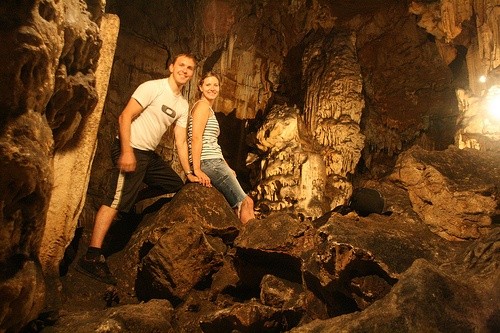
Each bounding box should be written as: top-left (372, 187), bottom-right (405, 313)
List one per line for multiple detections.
top-left (73, 254), bottom-right (118, 285)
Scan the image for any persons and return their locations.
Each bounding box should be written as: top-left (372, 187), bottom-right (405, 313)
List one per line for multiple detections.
top-left (75, 52), bottom-right (201, 284)
top-left (188, 72), bottom-right (255, 224)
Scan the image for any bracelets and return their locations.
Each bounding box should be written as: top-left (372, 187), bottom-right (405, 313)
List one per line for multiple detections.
top-left (185, 171), bottom-right (192, 176)
